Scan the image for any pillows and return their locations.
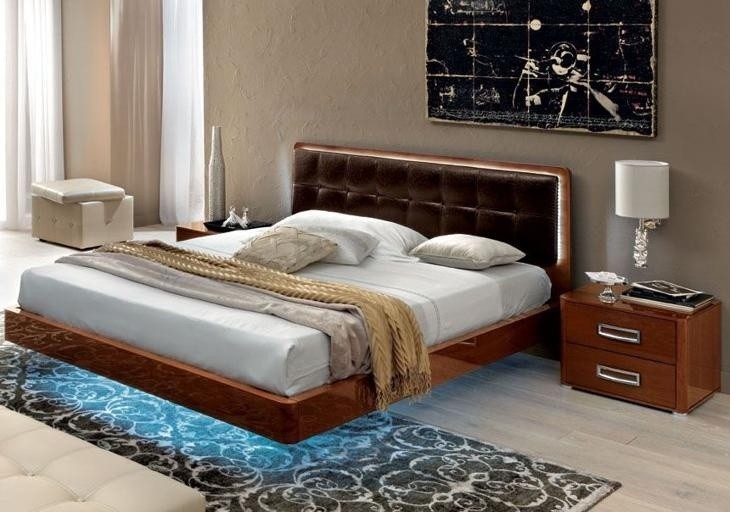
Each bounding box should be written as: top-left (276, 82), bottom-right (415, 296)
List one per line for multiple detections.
top-left (286, 224), bottom-right (378, 266)
top-left (233, 223), bottom-right (336, 274)
top-left (408, 233), bottom-right (527, 270)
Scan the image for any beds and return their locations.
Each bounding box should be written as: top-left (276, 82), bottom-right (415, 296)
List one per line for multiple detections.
top-left (4, 141), bottom-right (571, 444)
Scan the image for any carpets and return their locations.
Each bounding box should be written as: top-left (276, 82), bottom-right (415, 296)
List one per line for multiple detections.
top-left (0, 344), bottom-right (621, 512)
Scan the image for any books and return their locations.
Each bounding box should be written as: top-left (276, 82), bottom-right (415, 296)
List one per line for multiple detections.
top-left (619, 281), bottom-right (717, 316)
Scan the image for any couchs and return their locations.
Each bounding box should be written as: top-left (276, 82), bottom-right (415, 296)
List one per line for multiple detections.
top-left (31, 177), bottom-right (134, 250)
top-left (0, 403), bottom-right (208, 512)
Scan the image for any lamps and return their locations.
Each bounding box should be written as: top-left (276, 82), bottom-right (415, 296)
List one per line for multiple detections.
top-left (616, 160), bottom-right (669, 270)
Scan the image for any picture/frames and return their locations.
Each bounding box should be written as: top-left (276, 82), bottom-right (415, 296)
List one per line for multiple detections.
top-left (425, 2), bottom-right (658, 139)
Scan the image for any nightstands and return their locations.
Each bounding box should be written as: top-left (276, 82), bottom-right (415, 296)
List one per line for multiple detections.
top-left (562, 276), bottom-right (722, 417)
top-left (176, 221), bottom-right (222, 242)
